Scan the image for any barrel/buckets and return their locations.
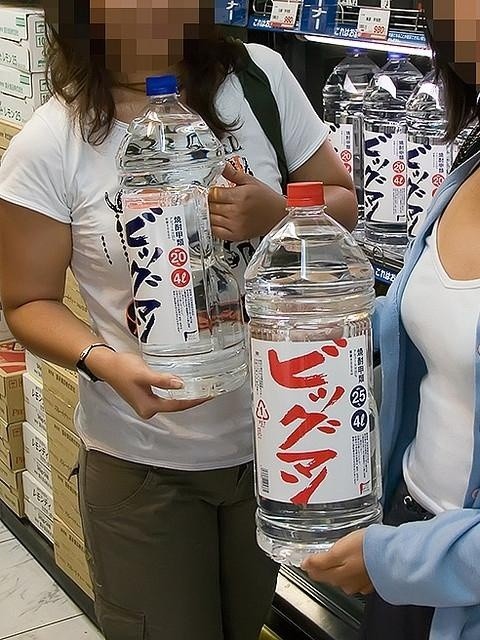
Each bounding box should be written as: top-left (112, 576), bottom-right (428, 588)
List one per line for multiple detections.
top-left (360, 50), bottom-right (423, 245)
top-left (239, 182), bottom-right (387, 570)
top-left (319, 47), bottom-right (380, 229)
top-left (404, 64), bottom-right (461, 253)
top-left (116, 74), bottom-right (248, 403)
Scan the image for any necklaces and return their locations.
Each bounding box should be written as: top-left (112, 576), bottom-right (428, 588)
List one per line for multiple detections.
top-left (115, 71), bottom-right (184, 96)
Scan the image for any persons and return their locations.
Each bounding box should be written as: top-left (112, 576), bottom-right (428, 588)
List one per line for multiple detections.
top-left (0, 0), bottom-right (360, 639)
top-left (300, 0), bottom-right (480, 638)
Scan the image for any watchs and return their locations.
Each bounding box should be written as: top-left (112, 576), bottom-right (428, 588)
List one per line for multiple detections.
top-left (73, 342), bottom-right (116, 385)
